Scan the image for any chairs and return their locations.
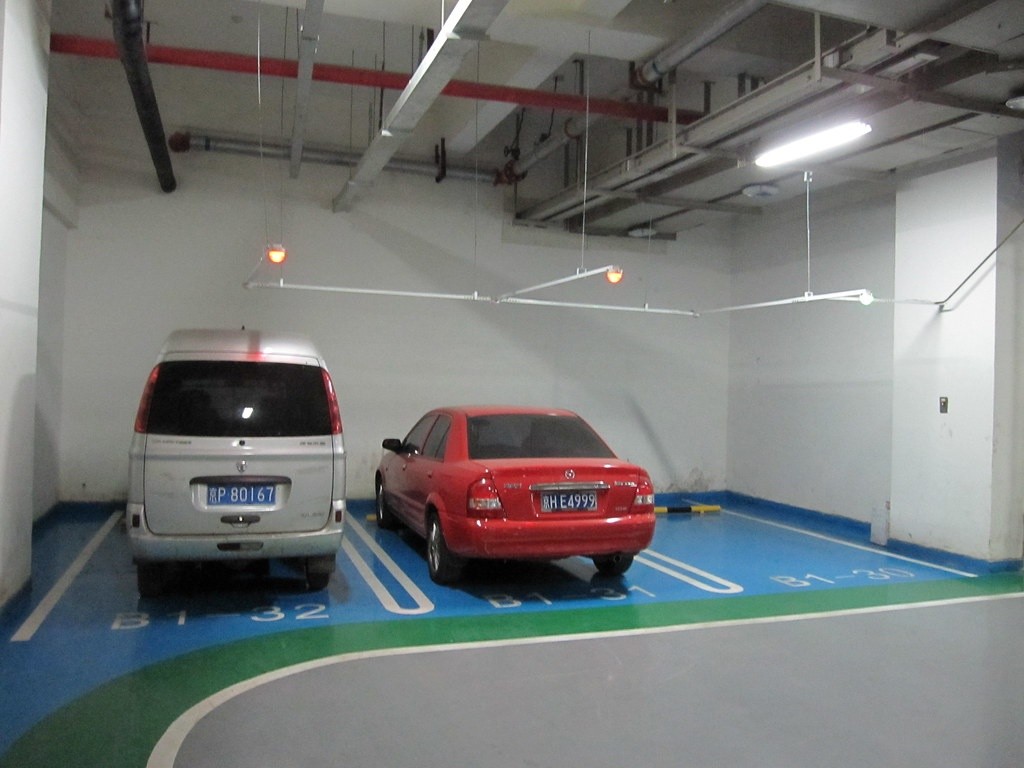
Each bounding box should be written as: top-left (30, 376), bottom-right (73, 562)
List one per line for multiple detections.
top-left (470, 443), bottom-right (527, 457)
top-left (231, 386), bottom-right (298, 435)
top-left (159, 388), bottom-right (226, 436)
top-left (469, 419), bottom-right (497, 444)
top-left (529, 417), bottom-right (563, 436)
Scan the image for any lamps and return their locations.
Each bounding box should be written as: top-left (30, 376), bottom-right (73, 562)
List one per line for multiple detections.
top-left (605, 266), bottom-right (622, 284)
top-left (754, 114), bottom-right (873, 168)
top-left (266, 244), bottom-right (287, 264)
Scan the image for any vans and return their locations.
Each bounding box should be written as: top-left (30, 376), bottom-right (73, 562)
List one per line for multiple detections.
top-left (126, 329), bottom-right (349, 594)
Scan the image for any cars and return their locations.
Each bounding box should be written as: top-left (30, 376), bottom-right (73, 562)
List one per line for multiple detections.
top-left (375, 404), bottom-right (658, 585)
